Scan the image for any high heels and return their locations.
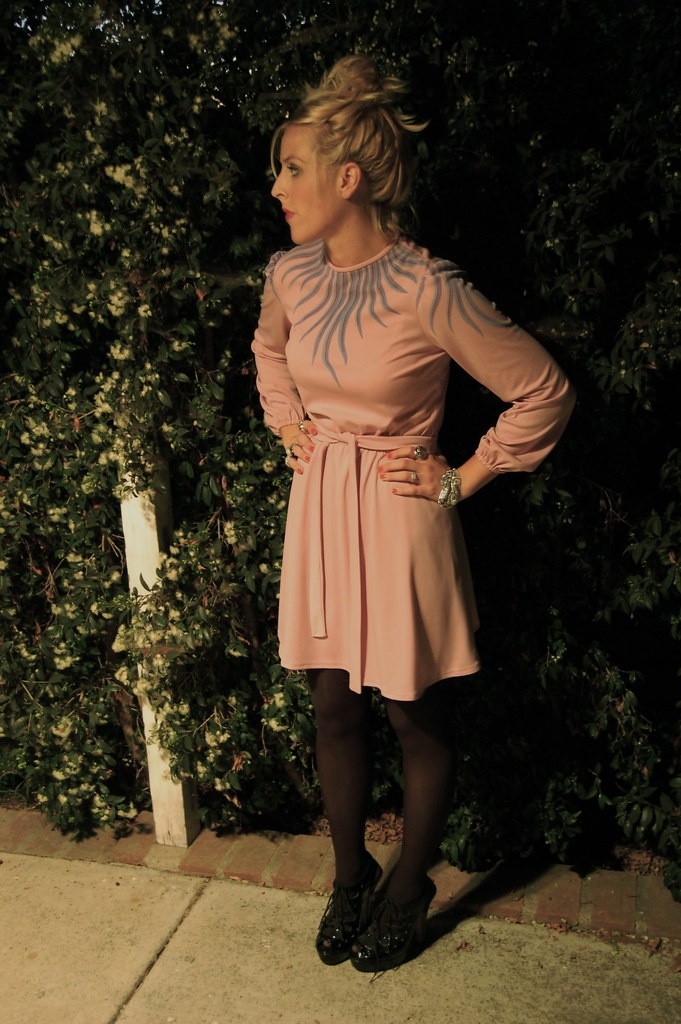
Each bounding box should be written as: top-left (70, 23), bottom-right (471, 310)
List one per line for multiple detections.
top-left (350, 877), bottom-right (436, 971)
top-left (316, 852), bottom-right (382, 965)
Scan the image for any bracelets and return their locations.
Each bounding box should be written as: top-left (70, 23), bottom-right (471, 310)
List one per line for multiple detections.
top-left (437, 468), bottom-right (461, 509)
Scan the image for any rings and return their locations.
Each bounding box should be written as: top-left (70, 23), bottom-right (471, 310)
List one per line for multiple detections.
top-left (411, 446), bottom-right (427, 460)
top-left (411, 472), bottom-right (417, 483)
top-left (285, 456), bottom-right (296, 467)
top-left (286, 444), bottom-right (302, 458)
top-left (298, 420), bottom-right (308, 433)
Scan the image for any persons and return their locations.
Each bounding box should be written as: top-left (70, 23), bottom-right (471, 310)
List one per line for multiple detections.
top-left (252, 55), bottom-right (573, 976)
